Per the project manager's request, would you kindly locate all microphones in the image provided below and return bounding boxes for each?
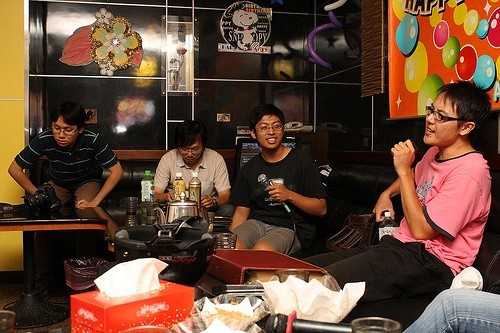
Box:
[264,311,353,333]
[258,174,291,212]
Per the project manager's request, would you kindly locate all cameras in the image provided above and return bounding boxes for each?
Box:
[20,183,62,214]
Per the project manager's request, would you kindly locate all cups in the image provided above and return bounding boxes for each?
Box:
[216,233,237,249]
[351,317,401,333]
[125,214,139,228]
[124,196,138,214]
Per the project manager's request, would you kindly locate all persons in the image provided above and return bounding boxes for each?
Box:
[301,80,492,305]
[154,120,236,217]
[228,104,328,256]
[402,288,500,333]
[8,105,123,295]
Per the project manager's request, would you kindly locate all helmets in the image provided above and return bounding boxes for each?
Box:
[114,216,215,287]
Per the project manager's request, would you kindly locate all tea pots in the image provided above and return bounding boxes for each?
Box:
[153,193,210,226]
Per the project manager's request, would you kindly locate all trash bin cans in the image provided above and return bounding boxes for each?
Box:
[65,258,108,299]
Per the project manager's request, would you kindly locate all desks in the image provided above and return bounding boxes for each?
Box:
[0,202,108,328]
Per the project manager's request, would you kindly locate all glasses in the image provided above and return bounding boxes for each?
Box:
[51,121,78,135]
[257,122,287,132]
[425,106,471,123]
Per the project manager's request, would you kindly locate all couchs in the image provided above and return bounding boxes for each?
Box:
[27,154,499,295]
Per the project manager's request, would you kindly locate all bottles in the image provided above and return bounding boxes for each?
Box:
[379,212,398,241]
[141,171,154,202]
[188,171,201,210]
[173,173,185,201]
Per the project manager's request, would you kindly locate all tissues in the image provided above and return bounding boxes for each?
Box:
[69,258,196,333]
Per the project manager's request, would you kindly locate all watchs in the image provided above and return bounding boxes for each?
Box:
[212,198,217,208]
